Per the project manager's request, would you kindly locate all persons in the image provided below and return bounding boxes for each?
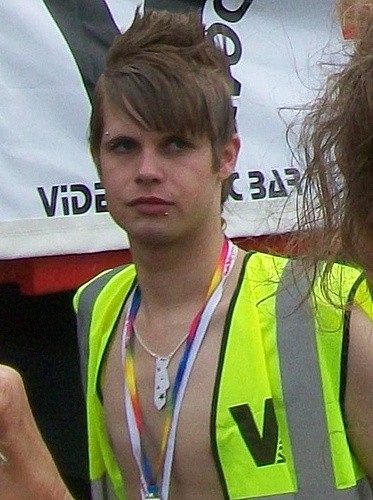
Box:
[0,0,373,500]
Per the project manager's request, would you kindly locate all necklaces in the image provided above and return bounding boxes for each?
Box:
[131,322,190,411]
